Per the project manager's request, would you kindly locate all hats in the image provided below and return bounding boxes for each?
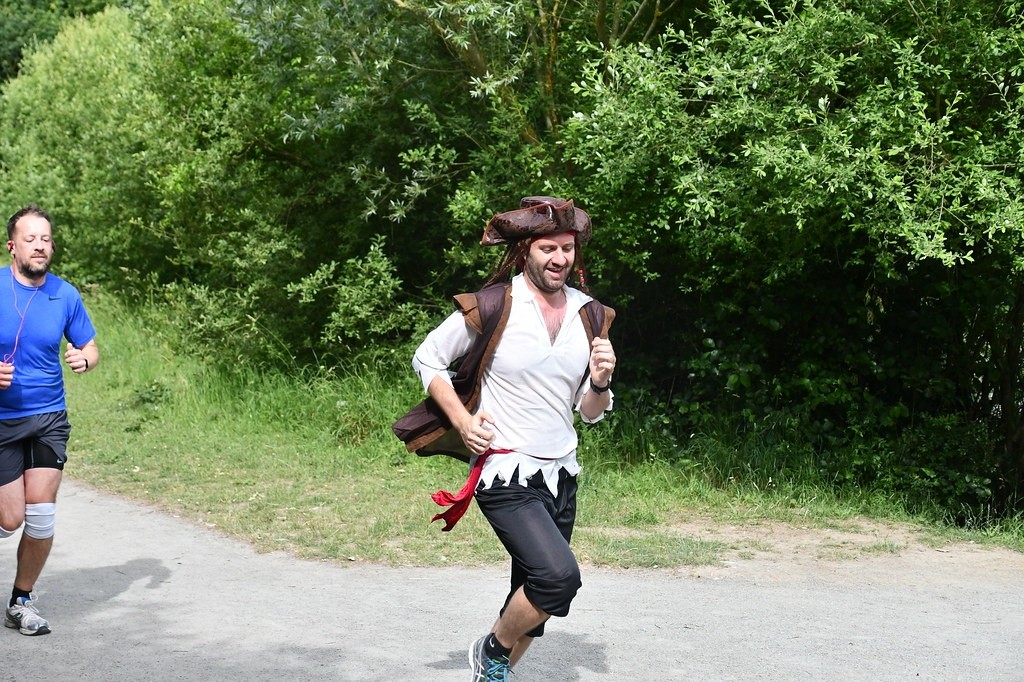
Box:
[479,196,592,247]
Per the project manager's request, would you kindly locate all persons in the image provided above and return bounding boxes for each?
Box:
[389,196,617,682]
[0,206,100,636]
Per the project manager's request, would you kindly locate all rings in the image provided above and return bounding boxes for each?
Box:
[477,438,481,446]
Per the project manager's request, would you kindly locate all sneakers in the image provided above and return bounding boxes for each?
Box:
[3,593,52,635]
[469,635,515,682]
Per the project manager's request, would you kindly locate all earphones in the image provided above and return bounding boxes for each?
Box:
[52,243,55,252]
[8,244,13,253]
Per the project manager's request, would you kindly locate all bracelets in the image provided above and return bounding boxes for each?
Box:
[589,376,611,394]
[83,358,88,372]
